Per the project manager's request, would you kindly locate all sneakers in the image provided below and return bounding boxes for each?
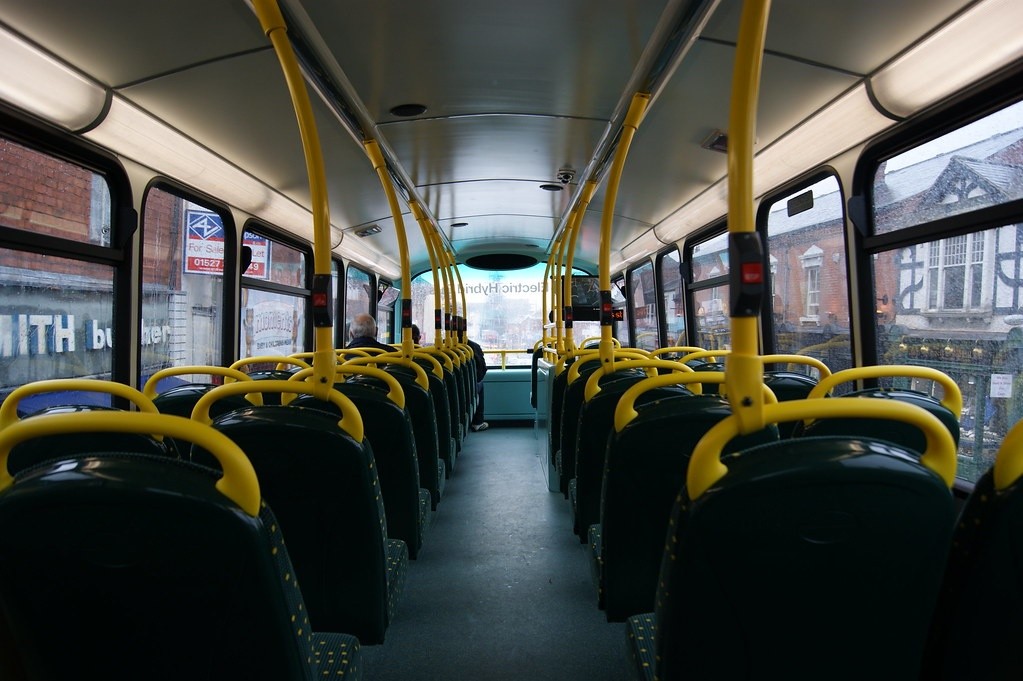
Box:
[471,422,488,433]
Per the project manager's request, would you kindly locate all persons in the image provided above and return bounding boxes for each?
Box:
[772,312,795,333]
[441,317,488,431]
[822,314,838,336]
[411,324,422,349]
[344,313,399,368]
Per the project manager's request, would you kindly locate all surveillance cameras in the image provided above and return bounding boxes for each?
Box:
[557,169,575,185]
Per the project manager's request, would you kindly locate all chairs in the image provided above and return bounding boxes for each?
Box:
[1,343,479,681]
[533,337,1023,680]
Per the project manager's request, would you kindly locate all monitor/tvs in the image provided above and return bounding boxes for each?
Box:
[562,275,600,308]
